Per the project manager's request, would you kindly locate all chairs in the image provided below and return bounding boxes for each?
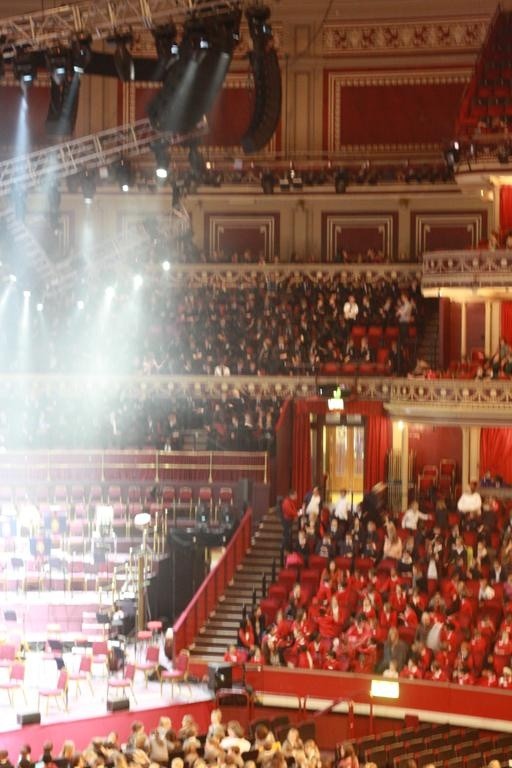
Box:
[170,283,421,375]
[228,554,511,691]
[283,500,509,558]
[0,481,232,592]
[417,461,456,499]
[0,611,193,717]
[427,347,512,382]
[338,723,512,767]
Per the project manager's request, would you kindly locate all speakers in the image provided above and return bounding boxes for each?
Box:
[147,45,232,132]
[208,663,231,689]
[241,48,281,154]
[107,698,129,710]
[46,79,80,135]
[17,712,40,724]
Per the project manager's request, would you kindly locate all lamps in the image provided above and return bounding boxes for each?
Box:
[445,138,510,171]
[1,245,176,318]
[165,161,452,194]
[10,133,208,221]
[1,2,274,93]
